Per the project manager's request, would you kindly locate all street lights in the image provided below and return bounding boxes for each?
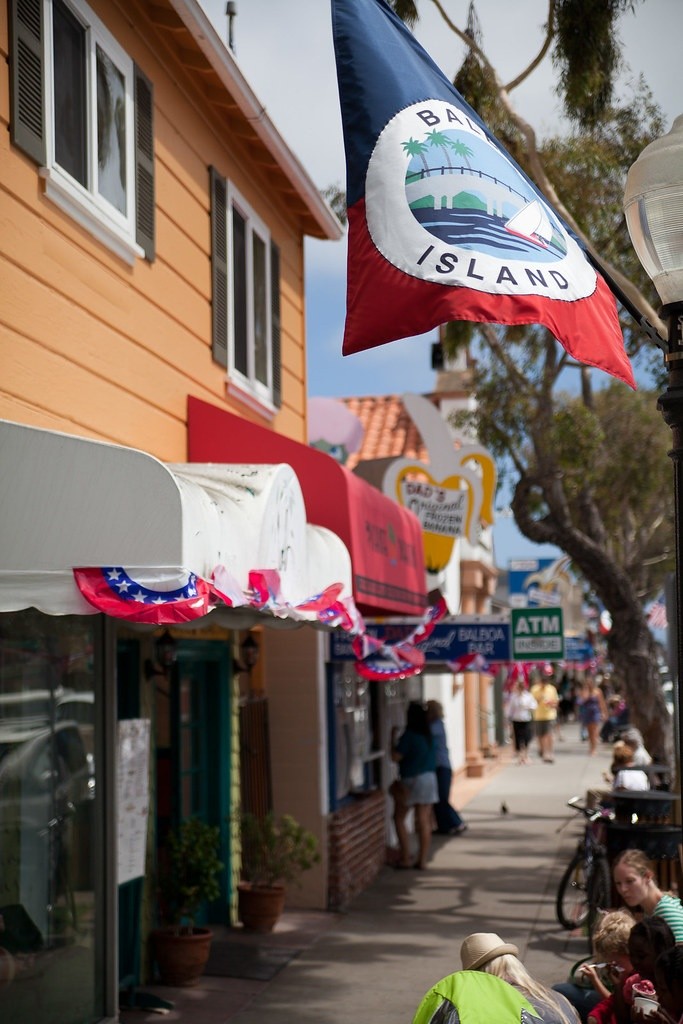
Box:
[624,108,683,900]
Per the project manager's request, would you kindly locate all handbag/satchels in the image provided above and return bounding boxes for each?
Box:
[389,780,412,801]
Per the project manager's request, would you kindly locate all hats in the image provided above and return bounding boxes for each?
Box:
[460,931,520,971]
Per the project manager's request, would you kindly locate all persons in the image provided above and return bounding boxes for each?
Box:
[588,908,636,1024]
[425,700,468,835]
[416,933,584,1024]
[616,916,675,1023]
[582,963,612,1002]
[587,728,659,825]
[507,666,629,763]
[631,945,683,1024]
[553,851,683,1022]
[391,700,439,871]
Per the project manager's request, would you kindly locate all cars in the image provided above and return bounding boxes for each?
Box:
[0,685,98,786]
[2,716,98,956]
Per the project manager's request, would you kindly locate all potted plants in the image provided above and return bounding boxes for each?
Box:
[236,810,324,937]
[149,817,229,989]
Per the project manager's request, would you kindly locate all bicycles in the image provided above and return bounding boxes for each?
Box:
[555,796,612,957]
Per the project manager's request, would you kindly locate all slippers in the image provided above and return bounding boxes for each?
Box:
[432,829,449,836]
[452,826,467,836]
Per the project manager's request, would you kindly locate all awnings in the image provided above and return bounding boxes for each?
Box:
[188,396,428,619]
[2,418,365,634]
[326,616,600,671]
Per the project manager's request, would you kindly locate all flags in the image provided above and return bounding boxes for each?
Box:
[332,1,640,390]
